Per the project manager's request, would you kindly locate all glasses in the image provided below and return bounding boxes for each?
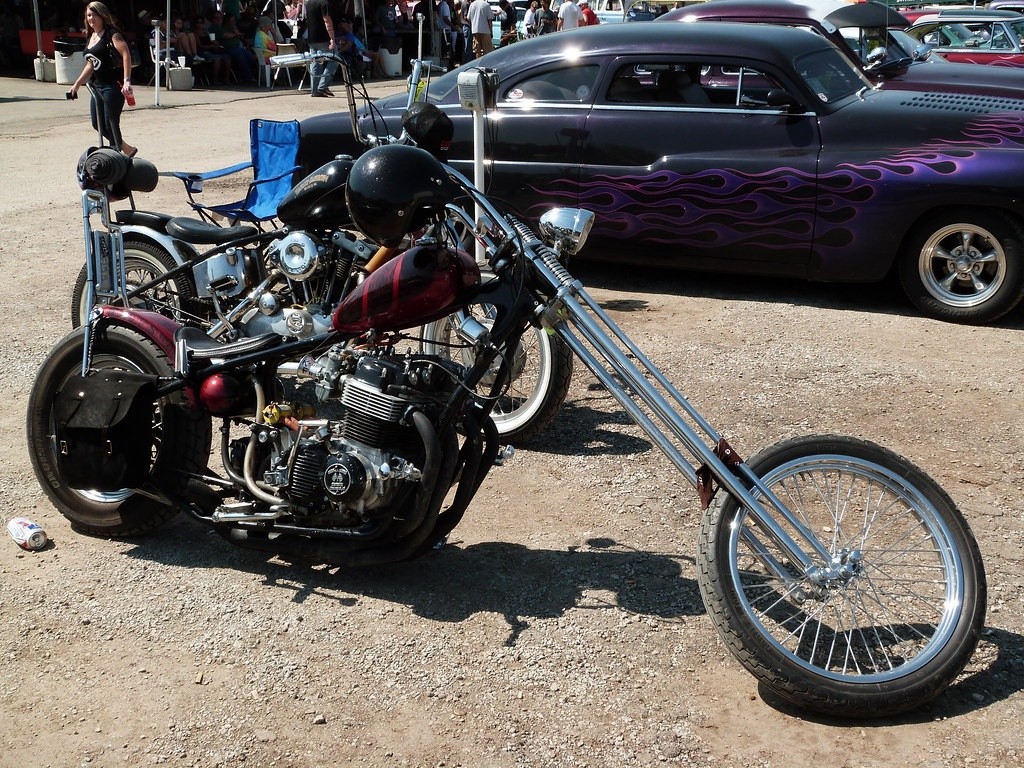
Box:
[195,21,203,24]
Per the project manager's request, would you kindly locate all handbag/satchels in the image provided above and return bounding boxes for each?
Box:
[52,369,159,492]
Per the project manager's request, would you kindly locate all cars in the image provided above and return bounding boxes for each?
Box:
[286,0,1024,326]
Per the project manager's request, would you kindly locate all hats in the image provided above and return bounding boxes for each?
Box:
[258,16,273,27]
[138,10,150,24]
[576,0,588,6]
[403,102,454,158]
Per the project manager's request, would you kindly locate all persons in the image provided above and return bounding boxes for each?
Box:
[492,0,519,48]
[306,0,405,97]
[69,1,138,158]
[138,0,293,86]
[522,0,600,39]
[412,0,496,72]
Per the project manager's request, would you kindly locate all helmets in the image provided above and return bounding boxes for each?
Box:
[345,144,452,248]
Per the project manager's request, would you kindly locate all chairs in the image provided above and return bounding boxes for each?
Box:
[252,47,292,87]
[658,71,710,104]
[609,78,642,99]
[173,117,305,235]
[147,46,179,86]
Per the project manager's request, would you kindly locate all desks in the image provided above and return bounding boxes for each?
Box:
[270,60,311,91]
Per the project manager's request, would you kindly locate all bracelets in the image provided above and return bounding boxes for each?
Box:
[123,78,131,80]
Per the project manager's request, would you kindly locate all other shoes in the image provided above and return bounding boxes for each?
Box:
[312,93,328,97]
[193,55,204,61]
[320,88,334,96]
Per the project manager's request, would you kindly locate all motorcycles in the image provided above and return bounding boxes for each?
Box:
[25,50,987,720]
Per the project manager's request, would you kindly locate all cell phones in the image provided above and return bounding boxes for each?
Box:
[66,92,78,99]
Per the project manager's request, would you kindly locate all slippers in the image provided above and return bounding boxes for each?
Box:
[129,147,138,158]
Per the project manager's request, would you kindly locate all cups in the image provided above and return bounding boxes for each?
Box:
[209,34,215,41]
[121,91,136,106]
[178,57,185,68]
[286,38,290,44]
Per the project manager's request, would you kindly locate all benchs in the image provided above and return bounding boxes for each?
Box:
[17,26,137,68]
